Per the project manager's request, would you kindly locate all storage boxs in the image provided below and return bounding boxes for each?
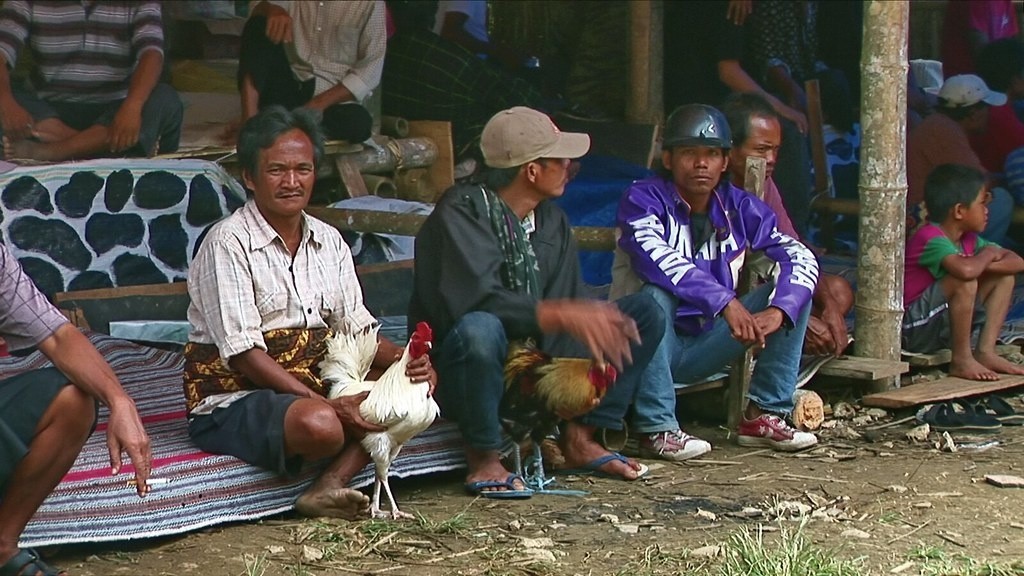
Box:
[172,15,246,58]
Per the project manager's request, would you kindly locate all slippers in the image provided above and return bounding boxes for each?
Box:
[465,473,533,498]
[970,394,1024,426]
[914,398,1004,434]
[560,452,650,482]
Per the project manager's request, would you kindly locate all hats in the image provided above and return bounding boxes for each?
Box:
[936,74,1009,108]
[479,105,592,170]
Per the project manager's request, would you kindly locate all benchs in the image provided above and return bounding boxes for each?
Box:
[0,58,750,547]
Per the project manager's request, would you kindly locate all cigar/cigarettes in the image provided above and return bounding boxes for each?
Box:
[127,478,174,485]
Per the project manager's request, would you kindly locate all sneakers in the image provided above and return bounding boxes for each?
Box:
[736,411,818,451]
[638,429,712,462]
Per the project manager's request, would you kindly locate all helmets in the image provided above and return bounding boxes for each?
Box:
[662,103,734,150]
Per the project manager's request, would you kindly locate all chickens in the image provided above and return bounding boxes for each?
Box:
[498,334,617,491]
[317,318,440,522]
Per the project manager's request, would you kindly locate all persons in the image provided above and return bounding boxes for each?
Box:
[0,0,611,164]
[609,107,854,461]
[0,240,151,576]
[183,104,437,519]
[408,107,642,497]
[701,0,1024,381]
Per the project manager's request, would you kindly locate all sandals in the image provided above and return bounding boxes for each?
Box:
[1,547,68,576]
